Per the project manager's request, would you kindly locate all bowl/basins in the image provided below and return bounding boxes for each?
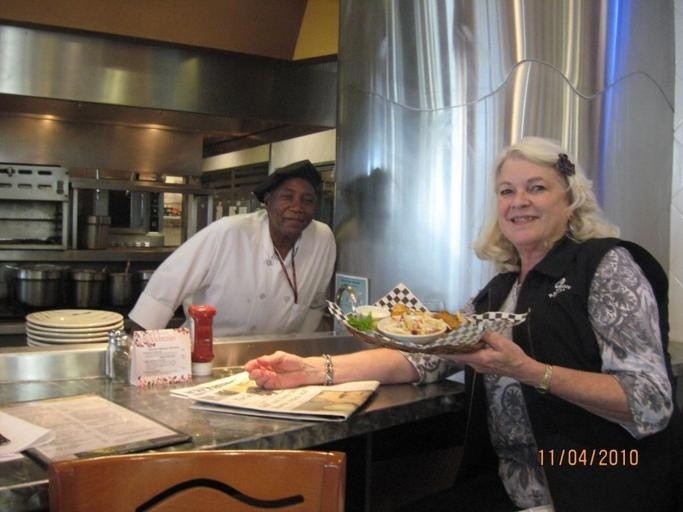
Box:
[375,315,448,344]
[354,305,390,322]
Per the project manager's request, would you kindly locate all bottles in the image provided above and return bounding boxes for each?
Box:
[187,303,216,378]
[104,328,130,391]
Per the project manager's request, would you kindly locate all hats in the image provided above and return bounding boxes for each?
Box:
[253,160,322,204]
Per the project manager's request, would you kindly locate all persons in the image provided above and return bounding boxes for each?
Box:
[128,158,338,338]
[245,136,683,511]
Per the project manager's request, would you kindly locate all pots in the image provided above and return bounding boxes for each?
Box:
[0,255,155,309]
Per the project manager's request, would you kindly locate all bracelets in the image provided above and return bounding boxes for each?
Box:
[323,354,333,385]
[535,364,553,394]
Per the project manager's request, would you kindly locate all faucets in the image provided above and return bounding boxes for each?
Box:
[334,283,358,314]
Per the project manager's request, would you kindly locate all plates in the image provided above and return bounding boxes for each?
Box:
[342,323,489,354]
[23,308,124,347]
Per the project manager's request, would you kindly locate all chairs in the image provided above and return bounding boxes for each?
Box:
[48,449,347,512]
